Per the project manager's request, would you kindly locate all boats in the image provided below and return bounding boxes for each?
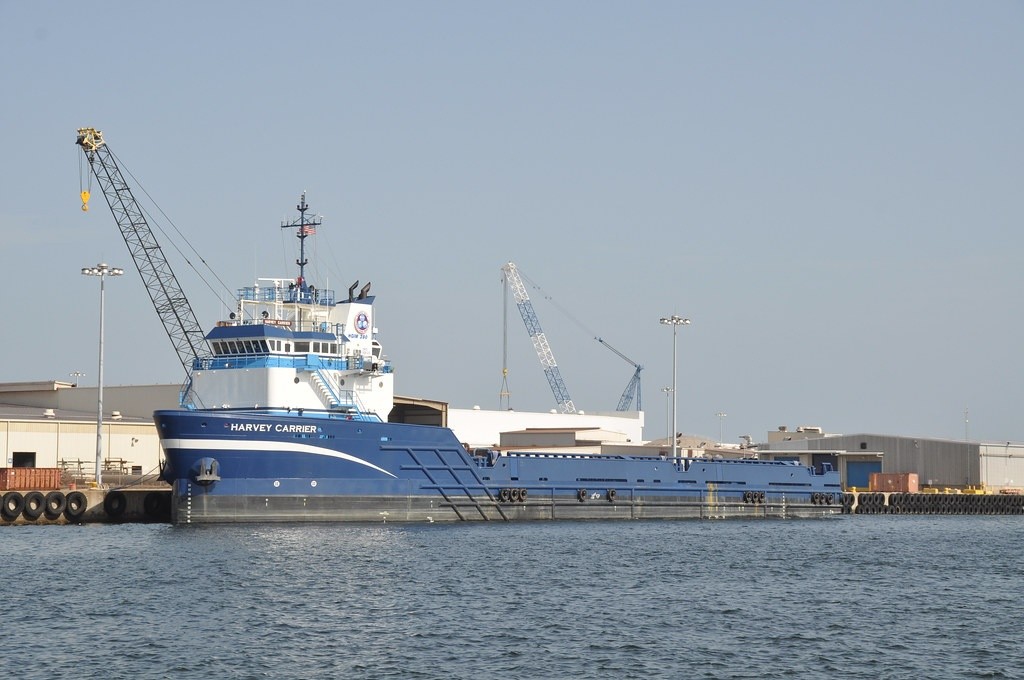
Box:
[153,190,854,527]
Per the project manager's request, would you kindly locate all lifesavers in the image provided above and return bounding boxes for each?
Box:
[742,490,766,503]
[499,488,528,502]
[103,490,128,518]
[840,492,1024,516]
[0,491,89,521]
[577,488,588,502]
[607,488,618,502]
[143,491,167,516]
[811,492,834,505]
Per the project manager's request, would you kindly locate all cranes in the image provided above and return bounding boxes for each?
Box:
[72,124,223,369]
[495,260,645,417]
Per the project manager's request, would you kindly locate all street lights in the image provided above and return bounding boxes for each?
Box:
[80,263,124,487]
[661,384,674,443]
[656,313,691,457]
[715,411,727,446]
